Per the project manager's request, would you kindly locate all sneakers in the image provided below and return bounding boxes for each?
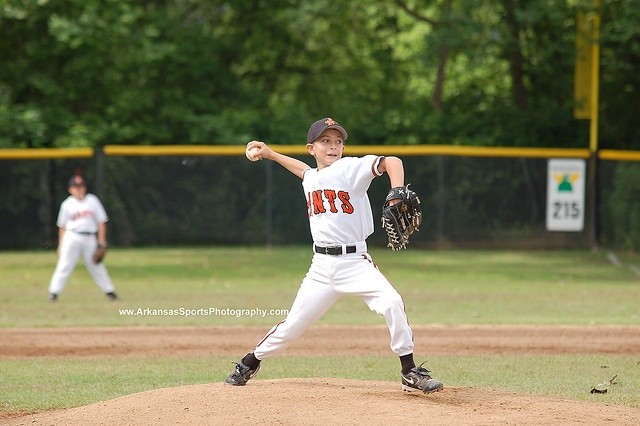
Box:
[47,294,58,304]
[402,360,444,394]
[224,353,260,387]
[107,292,119,302]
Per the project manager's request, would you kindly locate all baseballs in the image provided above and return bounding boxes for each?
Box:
[245,147,261,162]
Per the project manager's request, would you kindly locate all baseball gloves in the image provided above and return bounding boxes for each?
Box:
[94,244,106,263]
[382,183,422,251]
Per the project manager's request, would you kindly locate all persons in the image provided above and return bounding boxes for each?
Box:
[47,174,123,301]
[225,119,444,395]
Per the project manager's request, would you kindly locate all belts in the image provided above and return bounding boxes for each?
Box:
[72,232,98,235]
[312,245,358,256]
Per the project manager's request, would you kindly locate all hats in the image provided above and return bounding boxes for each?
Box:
[68,174,86,188]
[307,118,347,145]
